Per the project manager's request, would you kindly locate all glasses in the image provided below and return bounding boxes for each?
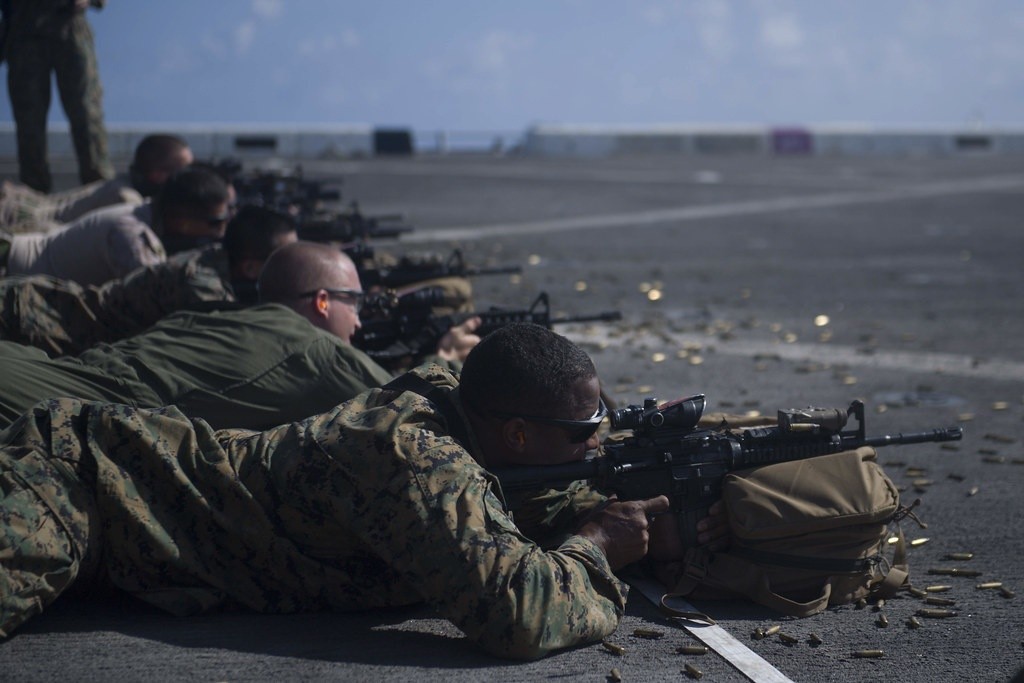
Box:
[299,289,365,313]
[180,211,230,231]
[489,395,606,444]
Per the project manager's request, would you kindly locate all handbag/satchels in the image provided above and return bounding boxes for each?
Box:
[599,404,916,619]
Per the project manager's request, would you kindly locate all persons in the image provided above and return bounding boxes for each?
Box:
[0,0,671,664]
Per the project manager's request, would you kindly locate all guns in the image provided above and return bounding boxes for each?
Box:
[350,286,621,373]
[293,207,415,245]
[342,243,524,291]
[486,391,965,558]
